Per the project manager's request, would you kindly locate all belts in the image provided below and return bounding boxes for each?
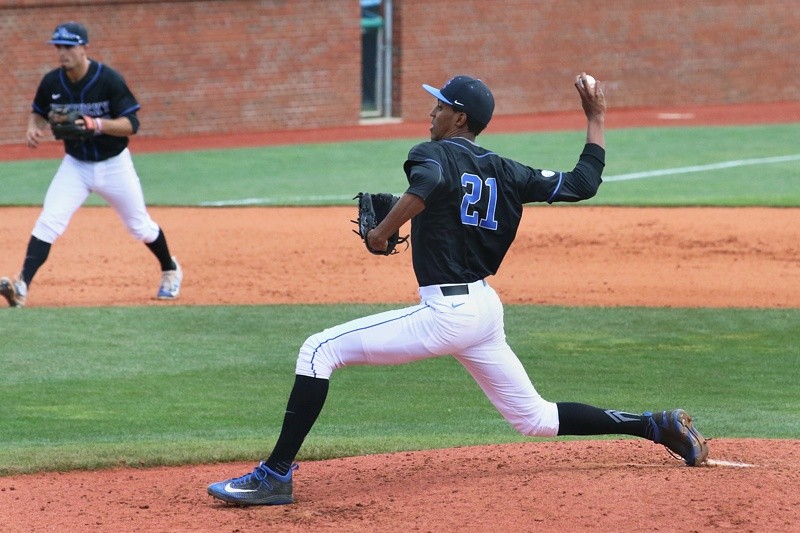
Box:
[441,280,488,295]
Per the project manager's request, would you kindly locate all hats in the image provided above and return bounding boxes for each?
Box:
[47,22,88,45]
[422,75,495,124]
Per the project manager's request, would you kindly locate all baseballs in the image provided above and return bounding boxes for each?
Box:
[579,75,596,89]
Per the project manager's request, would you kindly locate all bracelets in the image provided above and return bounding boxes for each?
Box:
[95,118,102,136]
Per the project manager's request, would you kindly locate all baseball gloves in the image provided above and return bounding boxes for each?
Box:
[48,111,97,146]
[349,192,410,257]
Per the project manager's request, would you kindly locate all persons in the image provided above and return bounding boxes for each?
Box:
[207,71,710,508]
[1,21,183,308]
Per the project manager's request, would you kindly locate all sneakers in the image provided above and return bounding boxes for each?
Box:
[651,409,709,467]
[0,277,27,308]
[157,256,182,298]
[207,462,299,504]
